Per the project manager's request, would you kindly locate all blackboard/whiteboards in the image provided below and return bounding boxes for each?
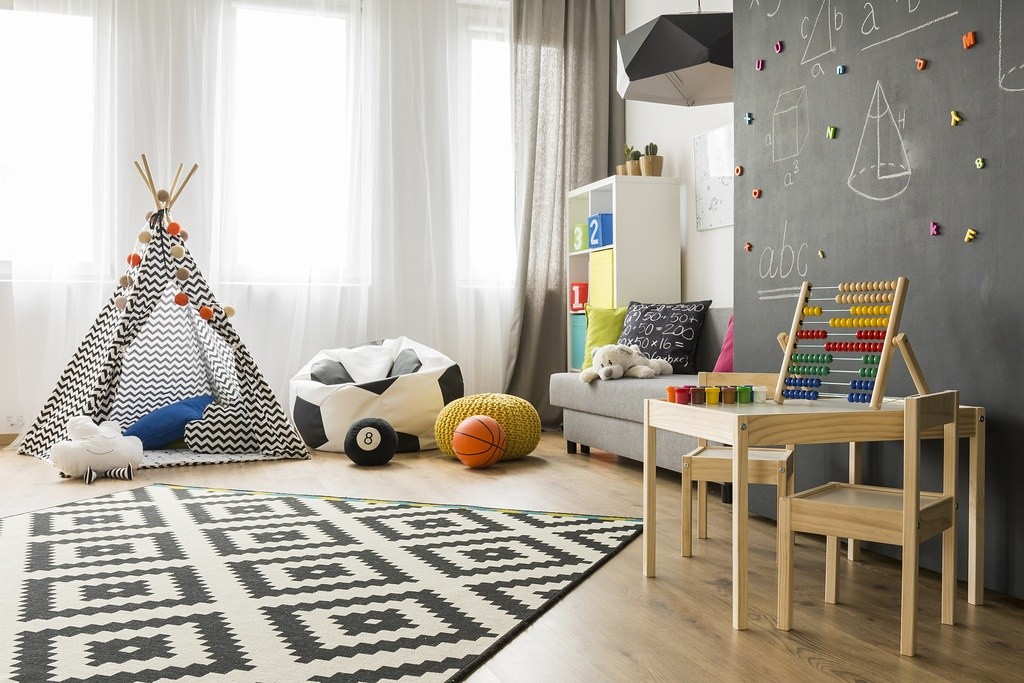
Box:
[692,122,734,231]
[733,0,1024,602]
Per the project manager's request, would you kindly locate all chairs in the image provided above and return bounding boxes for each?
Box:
[776,388,960,658]
[682,371,794,559]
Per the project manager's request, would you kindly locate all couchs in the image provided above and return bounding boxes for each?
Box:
[549,299,733,503]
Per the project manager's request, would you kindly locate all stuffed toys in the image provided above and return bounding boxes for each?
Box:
[49,416,144,484]
[579,344,672,385]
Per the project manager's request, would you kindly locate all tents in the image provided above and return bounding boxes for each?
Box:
[19,154,311,484]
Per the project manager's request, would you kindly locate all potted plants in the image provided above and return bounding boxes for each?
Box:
[615,145,635,175]
[626,151,641,175]
[640,143,664,176]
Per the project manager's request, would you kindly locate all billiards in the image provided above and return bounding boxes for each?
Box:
[343,419,397,467]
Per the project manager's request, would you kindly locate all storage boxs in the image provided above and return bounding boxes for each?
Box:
[573,224,588,252]
[570,282,588,311]
[587,212,611,248]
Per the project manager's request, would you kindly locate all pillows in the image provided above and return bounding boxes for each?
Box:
[581,302,627,371]
[617,299,712,374]
[123,394,216,449]
[712,310,733,372]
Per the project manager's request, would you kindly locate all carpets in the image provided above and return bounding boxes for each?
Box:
[0,482,658,683]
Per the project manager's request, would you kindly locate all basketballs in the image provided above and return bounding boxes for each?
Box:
[452,415,507,467]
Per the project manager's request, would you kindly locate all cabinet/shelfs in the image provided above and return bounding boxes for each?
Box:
[565,175,681,372]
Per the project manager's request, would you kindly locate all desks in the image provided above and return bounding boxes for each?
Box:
[642,394,985,632]
